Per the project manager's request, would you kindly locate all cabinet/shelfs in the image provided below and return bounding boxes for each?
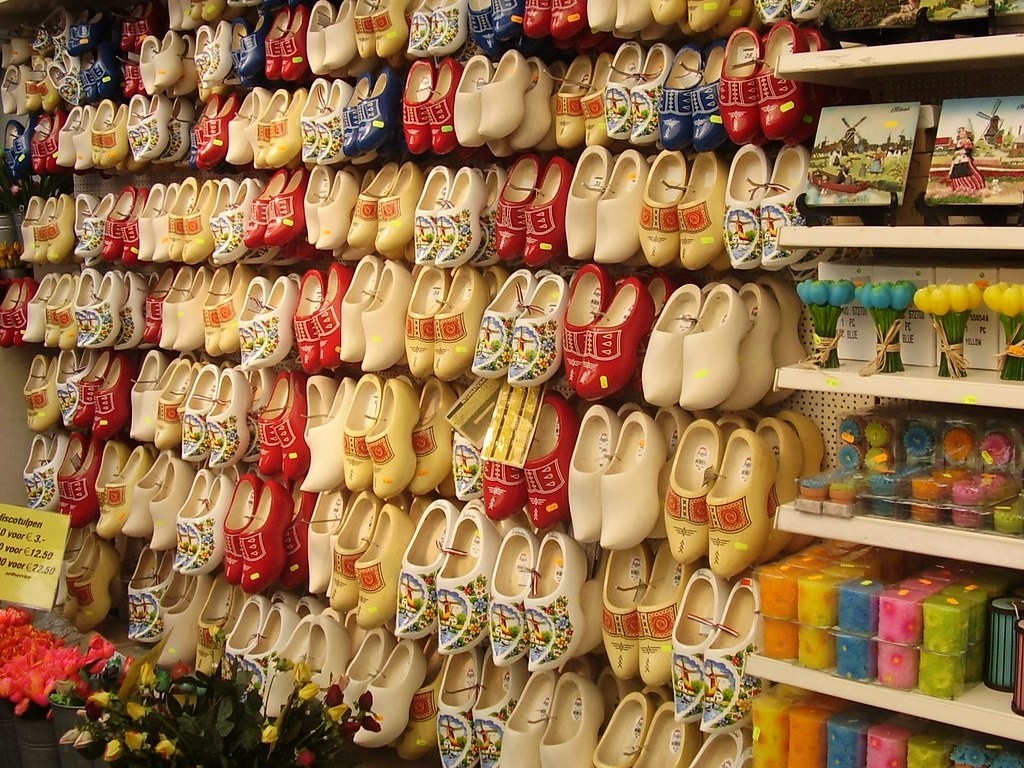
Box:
[736,29,1024,753]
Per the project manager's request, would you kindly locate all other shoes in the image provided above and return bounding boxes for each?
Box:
[0,0,826,768]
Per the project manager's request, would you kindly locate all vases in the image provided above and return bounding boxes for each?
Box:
[12,699,58,768]
[0,696,22,768]
[49,697,105,768]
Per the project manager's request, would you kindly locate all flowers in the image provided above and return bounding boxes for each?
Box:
[0,603,384,768]
